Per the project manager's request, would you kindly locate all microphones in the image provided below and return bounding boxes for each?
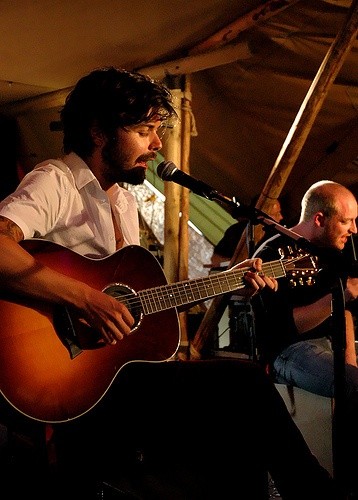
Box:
[157,160,218,201]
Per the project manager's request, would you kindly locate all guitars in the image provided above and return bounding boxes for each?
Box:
[0,239,322,424]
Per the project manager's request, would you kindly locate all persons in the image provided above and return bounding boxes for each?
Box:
[0,65,334,500]
[249,180,358,398]
[211,194,258,265]
